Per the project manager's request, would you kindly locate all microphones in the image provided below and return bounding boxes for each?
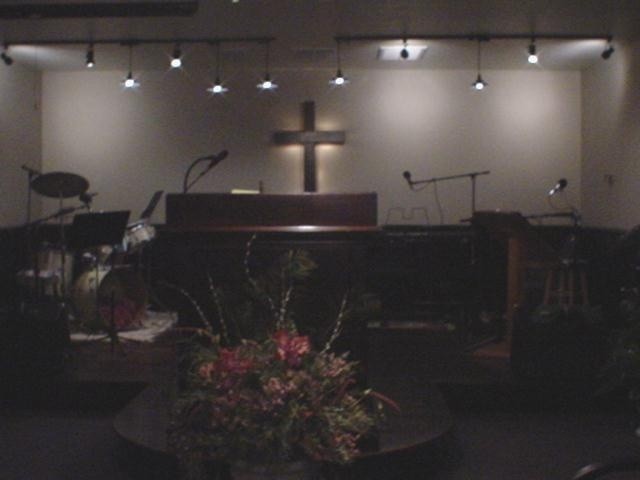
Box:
[403,171,414,189]
[259,180,264,194]
[21,164,41,176]
[79,191,93,209]
[548,178,568,196]
[208,150,229,168]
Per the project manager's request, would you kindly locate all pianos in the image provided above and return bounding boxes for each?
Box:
[155,193,383,353]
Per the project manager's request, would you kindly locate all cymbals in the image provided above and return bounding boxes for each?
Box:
[30,172,88,198]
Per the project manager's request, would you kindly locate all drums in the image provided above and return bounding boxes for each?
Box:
[125,219,155,250]
[70,267,136,331]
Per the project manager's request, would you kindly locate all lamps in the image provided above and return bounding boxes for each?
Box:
[0,35,282,93]
[324,30,617,91]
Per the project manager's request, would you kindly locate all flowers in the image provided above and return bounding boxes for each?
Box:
[171,327,379,467]
